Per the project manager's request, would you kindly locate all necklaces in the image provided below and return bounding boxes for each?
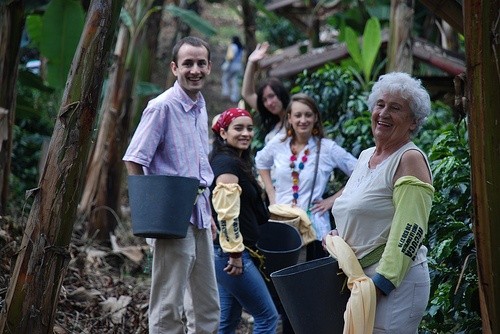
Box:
[290,146,310,205]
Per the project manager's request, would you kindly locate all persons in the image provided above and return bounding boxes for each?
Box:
[208,108,279,334]
[254,92,358,259]
[322,72,435,334]
[242,41,292,145]
[122,36,222,334]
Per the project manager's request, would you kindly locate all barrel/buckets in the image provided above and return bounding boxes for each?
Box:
[253,221,303,284]
[270,256,351,334]
[128,174,200,238]
